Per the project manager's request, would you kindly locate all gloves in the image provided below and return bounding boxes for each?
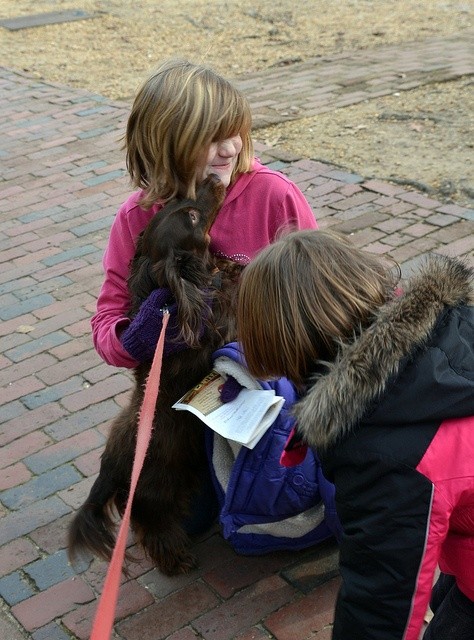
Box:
[219,375,244,403]
[120,286,216,363]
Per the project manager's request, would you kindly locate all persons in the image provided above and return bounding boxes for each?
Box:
[91,62,318,370]
[235,218,473,640]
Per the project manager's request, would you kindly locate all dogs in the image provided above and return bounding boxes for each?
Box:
[66,173,237,583]
